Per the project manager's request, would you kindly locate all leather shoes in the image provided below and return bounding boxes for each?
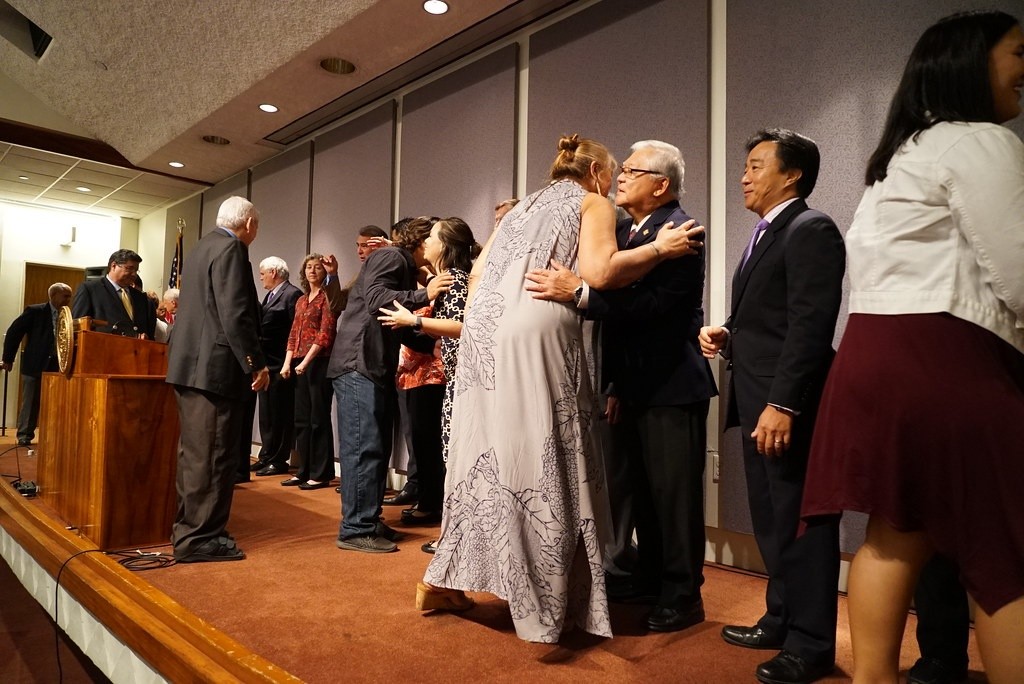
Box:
[754,650,834,683]
[401,508,417,517]
[256,462,289,475]
[299,480,329,490]
[401,511,441,525]
[422,540,437,553]
[281,479,306,486]
[382,490,415,505]
[642,595,706,633]
[721,625,782,649]
[605,580,660,605]
[250,460,268,470]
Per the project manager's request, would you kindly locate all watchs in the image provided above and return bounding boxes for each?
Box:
[573,287,584,303]
[412,317,423,335]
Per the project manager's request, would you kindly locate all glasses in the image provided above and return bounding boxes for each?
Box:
[620,165,661,176]
[115,261,141,275]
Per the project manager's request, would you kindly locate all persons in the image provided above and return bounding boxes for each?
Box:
[0,282,71,446]
[166,196,271,563]
[525,139,720,632]
[698,130,847,684]
[319,225,388,493]
[72,249,156,341]
[131,273,180,344]
[250,255,304,476]
[326,221,455,553]
[796,12,1024,684]
[280,253,336,490]
[493,198,520,229]
[366,217,483,554]
[416,134,705,644]
[599,192,638,578]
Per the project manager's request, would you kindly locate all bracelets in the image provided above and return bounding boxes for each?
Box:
[647,241,662,262]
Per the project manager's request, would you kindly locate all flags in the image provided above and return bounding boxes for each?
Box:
[168,232,185,289]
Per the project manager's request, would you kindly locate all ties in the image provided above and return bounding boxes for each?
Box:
[268,292,274,302]
[625,229,637,249]
[120,288,133,320]
[738,218,770,277]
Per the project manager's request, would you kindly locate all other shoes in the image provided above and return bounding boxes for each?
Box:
[179,537,245,562]
[336,533,398,553]
[904,662,968,683]
[375,521,404,541]
[18,439,32,446]
[234,473,250,484]
[335,486,341,493]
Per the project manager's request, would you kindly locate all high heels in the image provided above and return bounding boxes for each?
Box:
[415,583,474,611]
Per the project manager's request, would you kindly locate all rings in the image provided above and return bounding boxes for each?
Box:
[774,440,782,443]
